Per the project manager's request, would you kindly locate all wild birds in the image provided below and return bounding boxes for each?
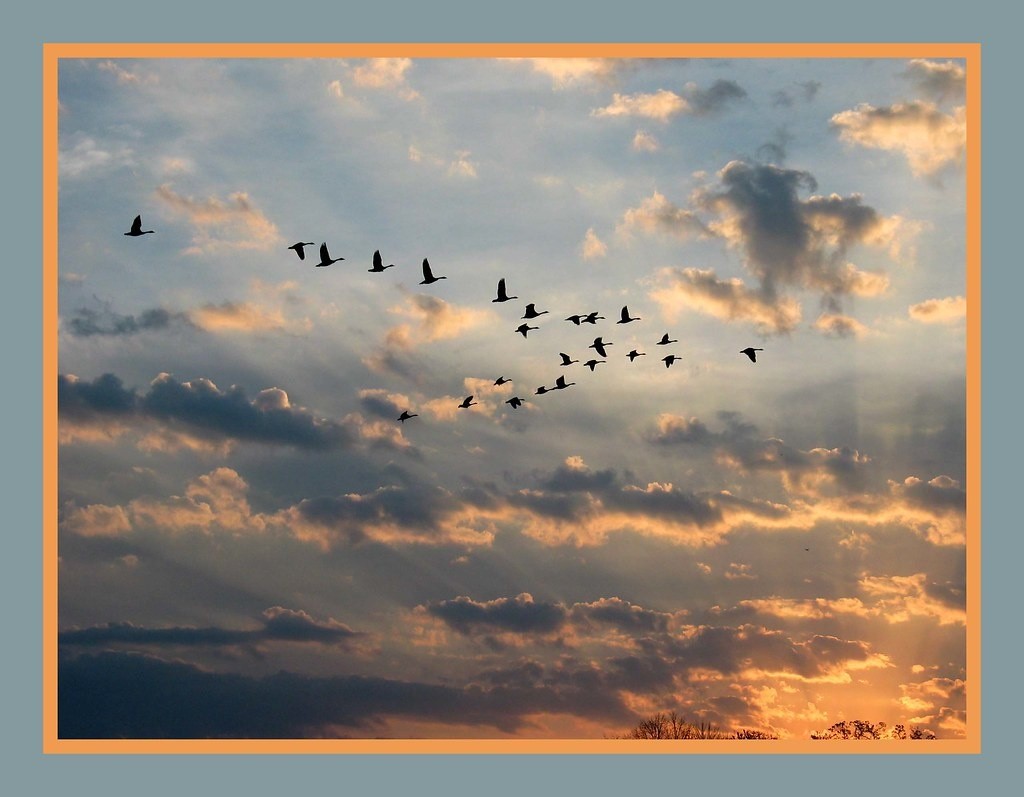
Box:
[492,277,764,371]
[124,215,155,237]
[288,242,447,285]
[397,375,576,425]
